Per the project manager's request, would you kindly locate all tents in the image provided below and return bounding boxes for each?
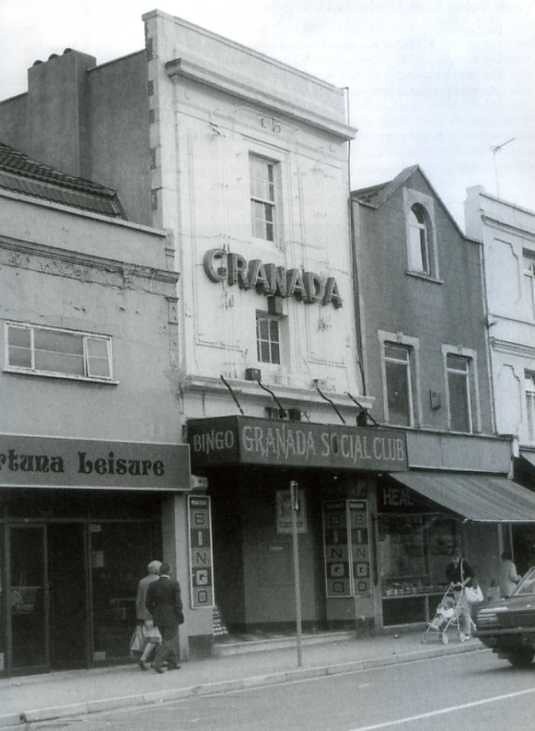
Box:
[388,470,534,526]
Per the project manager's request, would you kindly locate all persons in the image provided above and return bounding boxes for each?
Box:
[426,592,457,632]
[497,551,521,600]
[134,560,184,674]
[444,547,476,640]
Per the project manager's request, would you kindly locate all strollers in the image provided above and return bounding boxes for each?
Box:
[421,581,468,645]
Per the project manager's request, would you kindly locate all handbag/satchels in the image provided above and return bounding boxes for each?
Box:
[465,584,484,604]
[130,624,145,652]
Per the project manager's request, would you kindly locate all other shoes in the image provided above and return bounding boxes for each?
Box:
[168,664,180,670]
[151,663,164,673]
[464,635,470,640]
[139,659,148,670]
[160,663,166,667]
[472,624,477,631]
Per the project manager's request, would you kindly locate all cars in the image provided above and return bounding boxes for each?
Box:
[470,564,535,670]
